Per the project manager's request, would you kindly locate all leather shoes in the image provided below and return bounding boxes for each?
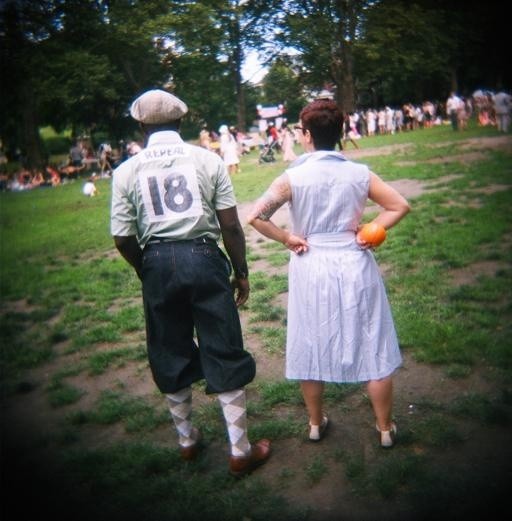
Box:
[177,428,204,462]
[228,438,272,478]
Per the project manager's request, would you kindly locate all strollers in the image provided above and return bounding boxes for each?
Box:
[256,139,279,163]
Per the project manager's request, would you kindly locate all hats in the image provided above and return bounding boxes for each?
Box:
[130,88,189,125]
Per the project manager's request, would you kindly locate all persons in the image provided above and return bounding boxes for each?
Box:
[246,99,413,449]
[107,90,271,473]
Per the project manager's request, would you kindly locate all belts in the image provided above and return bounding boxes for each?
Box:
[145,233,217,247]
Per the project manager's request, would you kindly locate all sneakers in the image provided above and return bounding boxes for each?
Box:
[308,416,328,443]
[375,418,399,449]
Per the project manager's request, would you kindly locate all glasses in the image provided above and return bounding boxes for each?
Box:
[292,124,305,131]
